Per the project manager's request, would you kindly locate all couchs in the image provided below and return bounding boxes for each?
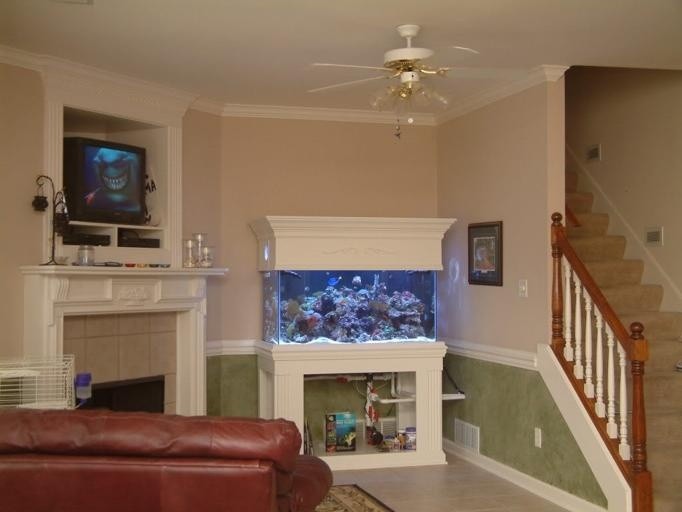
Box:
[1,407,333,512]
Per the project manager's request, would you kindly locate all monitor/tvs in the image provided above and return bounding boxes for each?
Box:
[63,137,145,225]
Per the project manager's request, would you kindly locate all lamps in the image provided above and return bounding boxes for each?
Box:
[369,69,450,139]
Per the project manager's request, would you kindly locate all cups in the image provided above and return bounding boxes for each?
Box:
[183,232,216,268]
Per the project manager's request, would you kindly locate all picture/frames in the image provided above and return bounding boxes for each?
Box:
[468,221,502,286]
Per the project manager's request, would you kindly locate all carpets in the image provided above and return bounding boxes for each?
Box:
[315,483,395,512]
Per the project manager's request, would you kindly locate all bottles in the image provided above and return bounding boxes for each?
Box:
[390,437,401,454]
[77,243,95,266]
[406,427,416,451]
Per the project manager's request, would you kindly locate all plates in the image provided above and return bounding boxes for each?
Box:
[125,263,171,268]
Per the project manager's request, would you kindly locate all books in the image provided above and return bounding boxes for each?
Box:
[326,412,357,452]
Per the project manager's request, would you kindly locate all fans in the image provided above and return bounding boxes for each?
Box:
[307,24,515,99]
